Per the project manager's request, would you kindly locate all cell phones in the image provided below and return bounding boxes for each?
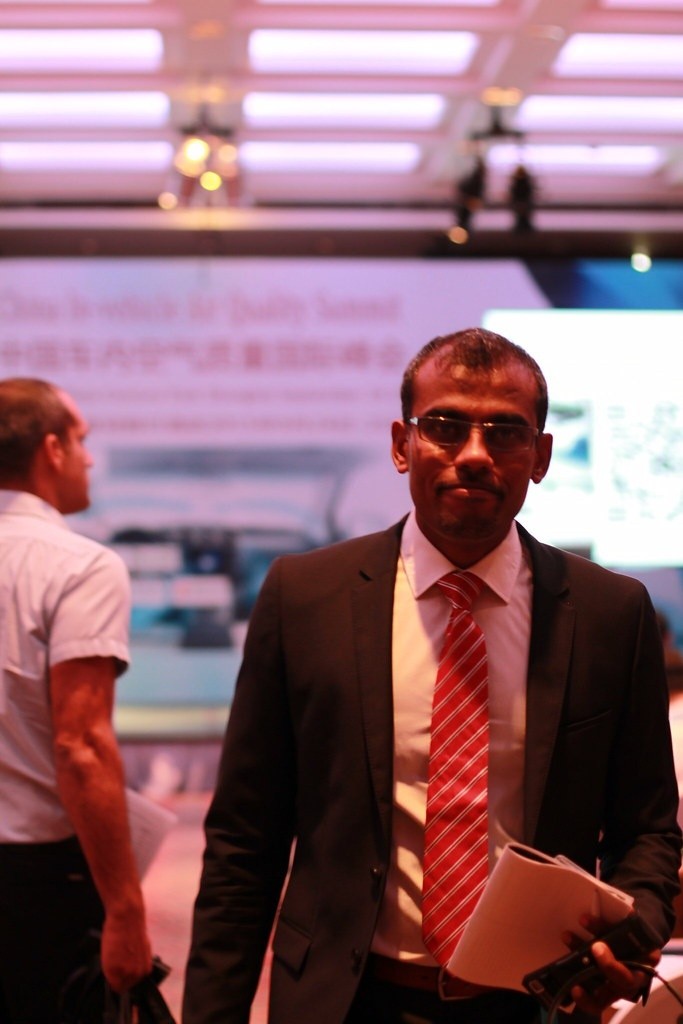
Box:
[523,914,664,1012]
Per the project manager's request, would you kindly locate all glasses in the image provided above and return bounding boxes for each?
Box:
[403,415,541,454]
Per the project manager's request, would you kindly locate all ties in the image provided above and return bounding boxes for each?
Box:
[420,572,492,977]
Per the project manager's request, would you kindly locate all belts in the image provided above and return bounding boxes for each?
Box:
[363,951,497,1001]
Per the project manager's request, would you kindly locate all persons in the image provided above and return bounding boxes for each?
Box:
[184,328,683,1024]
[0,376,179,1024]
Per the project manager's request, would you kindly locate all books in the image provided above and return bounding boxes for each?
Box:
[443,840,633,994]
